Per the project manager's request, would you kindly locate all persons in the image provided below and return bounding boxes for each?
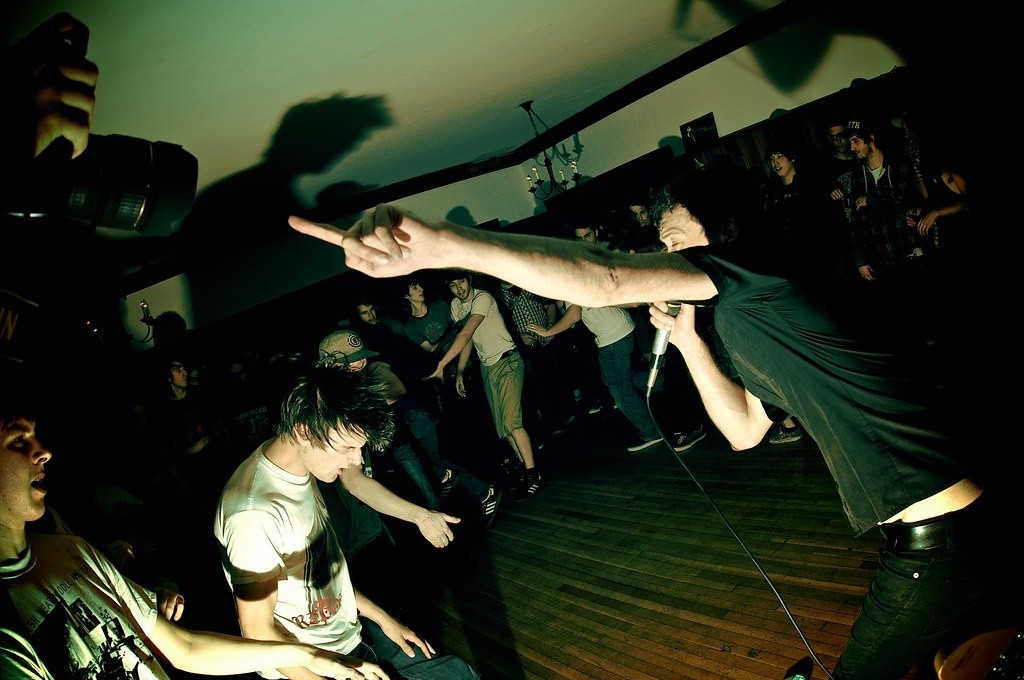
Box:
[0,329,392,680]
[96,101,992,621]
[213,329,482,680]
[288,182,1024,680]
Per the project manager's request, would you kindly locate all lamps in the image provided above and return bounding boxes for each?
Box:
[517,100,583,200]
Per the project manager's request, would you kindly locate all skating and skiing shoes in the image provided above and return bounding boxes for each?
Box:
[623,433,663,451]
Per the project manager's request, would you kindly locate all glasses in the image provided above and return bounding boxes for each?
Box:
[900,210,922,228]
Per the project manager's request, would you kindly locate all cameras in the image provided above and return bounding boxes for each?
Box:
[0,117,199,237]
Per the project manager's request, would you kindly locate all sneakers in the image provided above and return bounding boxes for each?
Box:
[511,466,543,502]
[481,485,502,530]
[674,422,706,452]
[766,425,801,445]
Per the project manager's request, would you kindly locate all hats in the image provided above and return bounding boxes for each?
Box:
[313,329,382,370]
[831,115,879,142]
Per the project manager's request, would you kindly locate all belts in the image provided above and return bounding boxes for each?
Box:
[499,349,514,360]
[867,498,999,549]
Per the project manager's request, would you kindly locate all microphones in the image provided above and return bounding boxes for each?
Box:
[645,301,683,398]
